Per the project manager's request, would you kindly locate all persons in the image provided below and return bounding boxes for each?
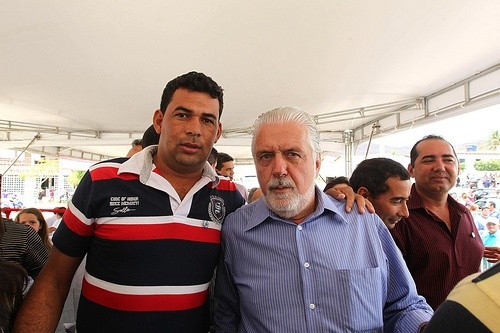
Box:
[349,158,412,229]
[390,135,486,311]
[215,106,435,333]
[0,124,264,333]
[423,261,500,333]
[446,171,500,263]
[10,72,374,333]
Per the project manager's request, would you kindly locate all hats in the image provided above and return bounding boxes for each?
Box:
[485,217,499,225]
